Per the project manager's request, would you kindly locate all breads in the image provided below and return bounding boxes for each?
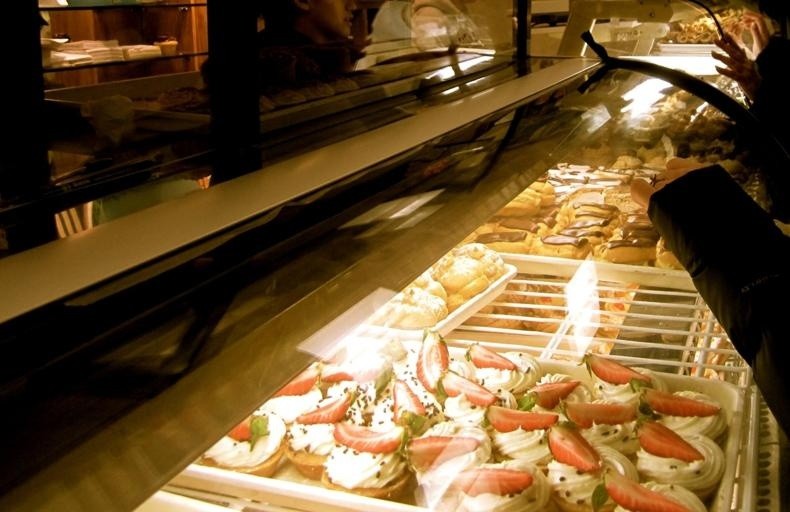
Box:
[158,65,401,112]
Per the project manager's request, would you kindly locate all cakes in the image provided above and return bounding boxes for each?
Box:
[583,92,744,181]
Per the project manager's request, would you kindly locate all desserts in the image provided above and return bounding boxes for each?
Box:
[372,161,749,329]
[203,333,727,512]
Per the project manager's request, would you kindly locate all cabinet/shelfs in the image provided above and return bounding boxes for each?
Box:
[0,0,790,512]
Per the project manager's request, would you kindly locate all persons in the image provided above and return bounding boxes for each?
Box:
[197,1,375,138]
[633,157,790,446]
[401,0,485,100]
[713,2,790,233]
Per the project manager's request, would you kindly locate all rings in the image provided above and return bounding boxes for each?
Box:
[649,174,664,188]
